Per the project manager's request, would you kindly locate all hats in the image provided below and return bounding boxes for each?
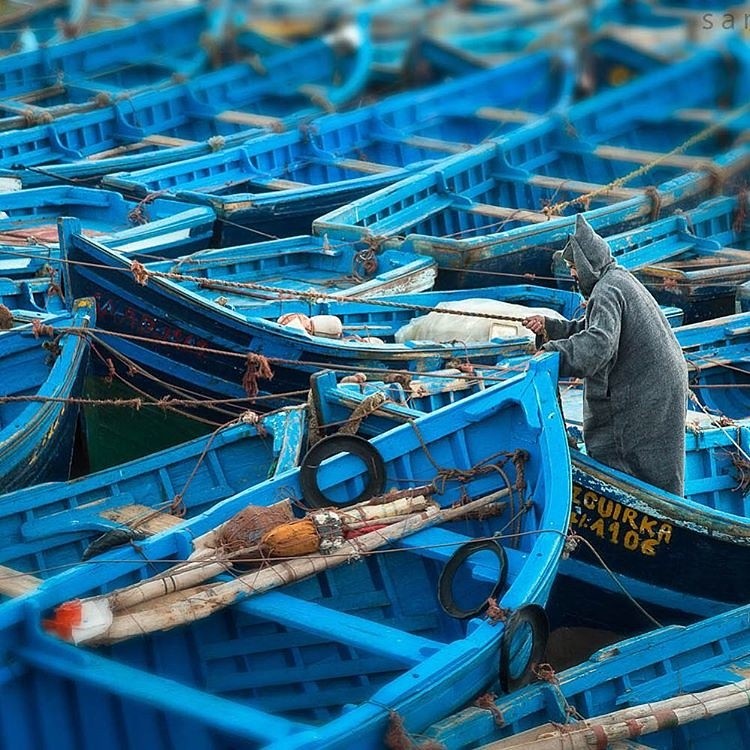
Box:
[561,241,574,262]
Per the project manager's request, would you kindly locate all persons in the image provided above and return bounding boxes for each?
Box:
[522,213,689,498]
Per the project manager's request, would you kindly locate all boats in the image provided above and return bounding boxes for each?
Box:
[0,2,750,750]
